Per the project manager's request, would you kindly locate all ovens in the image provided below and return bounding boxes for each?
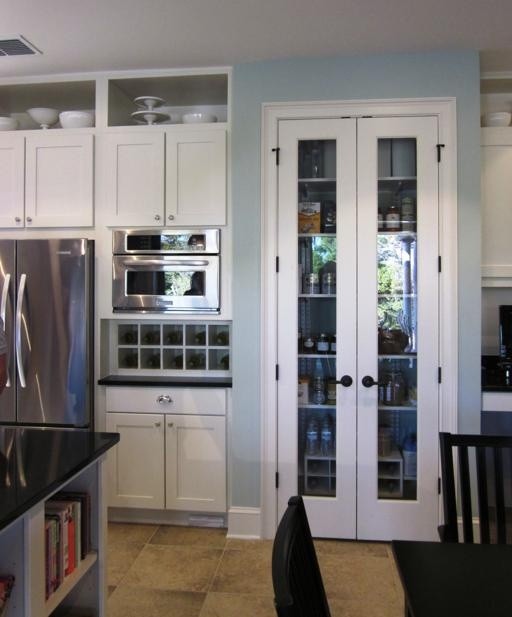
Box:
[111,226,221,316]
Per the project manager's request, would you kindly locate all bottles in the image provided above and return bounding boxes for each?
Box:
[165,331,183,346]
[321,271,336,294]
[122,353,138,368]
[220,355,229,370]
[307,415,336,457]
[172,354,183,368]
[216,330,229,345]
[302,273,320,293]
[194,331,205,345]
[188,353,205,369]
[120,330,138,344]
[142,331,160,345]
[147,354,160,368]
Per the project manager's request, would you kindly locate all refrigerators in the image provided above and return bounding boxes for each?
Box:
[0,239,96,428]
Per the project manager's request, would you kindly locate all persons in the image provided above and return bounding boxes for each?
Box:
[184,271,204,296]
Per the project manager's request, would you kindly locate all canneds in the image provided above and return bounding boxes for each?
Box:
[330,334,336,354]
[303,337,315,352]
[313,375,325,404]
[316,333,329,354]
[326,378,336,405]
[384,205,401,231]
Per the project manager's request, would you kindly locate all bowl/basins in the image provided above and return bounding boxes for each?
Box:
[0,116,18,130]
[58,109,95,128]
[182,113,218,123]
[10,112,34,129]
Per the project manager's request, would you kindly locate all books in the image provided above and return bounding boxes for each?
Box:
[46,491,93,601]
[0,575,16,617]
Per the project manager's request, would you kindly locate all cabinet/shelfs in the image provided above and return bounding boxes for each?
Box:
[0,452,109,617]
[102,386,230,529]
[260,97,459,545]
[0,72,100,239]
[107,320,232,377]
[102,65,233,230]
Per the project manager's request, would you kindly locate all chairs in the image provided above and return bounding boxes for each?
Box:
[272,496,332,617]
[438,432,512,543]
